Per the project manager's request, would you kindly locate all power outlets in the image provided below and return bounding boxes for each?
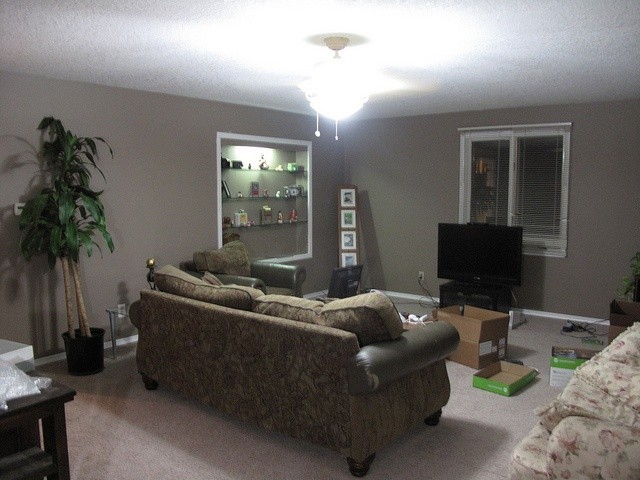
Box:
[419,272,424,281]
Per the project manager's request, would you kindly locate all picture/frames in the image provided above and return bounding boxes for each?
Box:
[338,183,360,272]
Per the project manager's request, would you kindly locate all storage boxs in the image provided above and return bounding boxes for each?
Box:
[432,305,510,368]
[550,346,599,388]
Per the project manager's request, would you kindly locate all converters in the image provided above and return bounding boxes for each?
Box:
[563,322,574,333]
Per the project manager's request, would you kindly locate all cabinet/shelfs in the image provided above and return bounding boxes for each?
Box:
[0,351,76,480]
[215,130,313,262]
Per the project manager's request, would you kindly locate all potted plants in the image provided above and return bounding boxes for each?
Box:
[6,121,111,371]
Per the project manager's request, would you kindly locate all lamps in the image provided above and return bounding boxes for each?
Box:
[293,34,370,140]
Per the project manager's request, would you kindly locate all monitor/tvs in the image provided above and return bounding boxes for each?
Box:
[327,264,364,298]
[436,222,523,288]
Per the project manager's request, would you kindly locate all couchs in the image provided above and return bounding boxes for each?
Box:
[129,263,459,477]
[509,323,640,479]
[180,241,306,298]
